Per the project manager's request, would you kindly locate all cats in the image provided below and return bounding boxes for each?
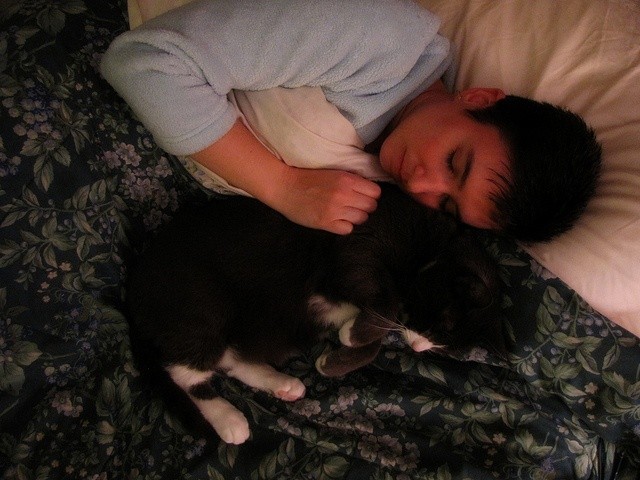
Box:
[122,183,501,445]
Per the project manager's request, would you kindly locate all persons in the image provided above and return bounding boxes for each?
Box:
[101,0,601,246]
